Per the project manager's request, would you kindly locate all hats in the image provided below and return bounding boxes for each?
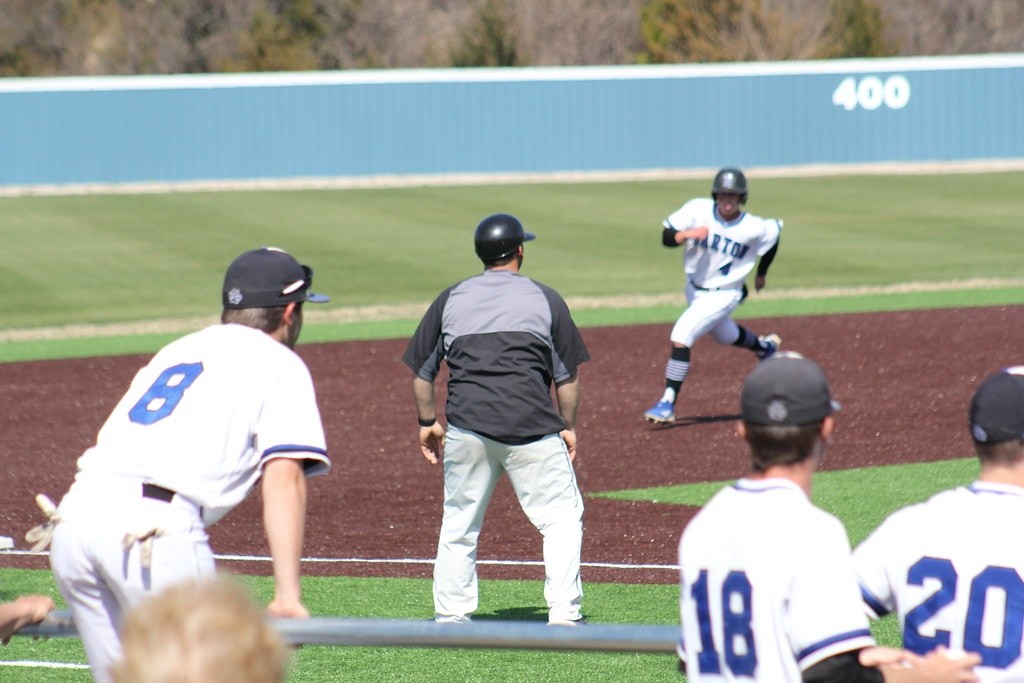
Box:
[970,364,1024,439]
[741,351,841,425]
[474,214,536,260]
[711,169,748,204]
[222,246,329,309]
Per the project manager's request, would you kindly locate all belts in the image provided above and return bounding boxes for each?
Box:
[690,280,743,292]
[69,476,203,521]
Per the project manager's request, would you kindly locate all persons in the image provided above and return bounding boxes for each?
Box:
[644,168,781,425]
[679,350,985,683]
[400,213,591,628]
[850,364,1024,683]
[33,244,331,683]
[0,595,57,645]
[107,575,292,683]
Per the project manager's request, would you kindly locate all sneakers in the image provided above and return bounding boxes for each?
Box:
[643,403,678,425]
[756,332,782,367]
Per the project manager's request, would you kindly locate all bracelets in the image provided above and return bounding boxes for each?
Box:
[418,415,436,427]
